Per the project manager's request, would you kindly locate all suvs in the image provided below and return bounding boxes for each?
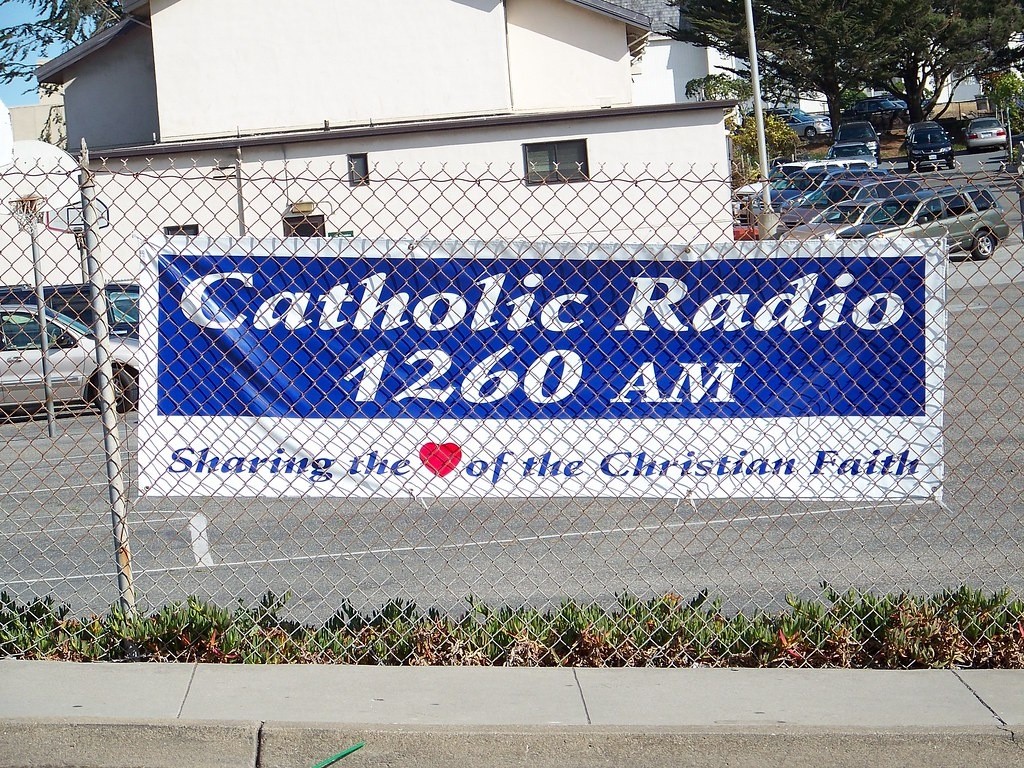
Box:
[775,180,925,236]
[731,157,873,214]
[740,167,887,224]
[836,182,1010,260]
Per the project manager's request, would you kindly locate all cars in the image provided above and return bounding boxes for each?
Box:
[1,284,141,338]
[779,197,888,240]
[836,95,936,125]
[905,121,945,140]
[0,305,139,413]
[904,128,957,171]
[835,121,884,165]
[825,142,879,173]
[743,106,832,137]
[960,117,1009,150]
